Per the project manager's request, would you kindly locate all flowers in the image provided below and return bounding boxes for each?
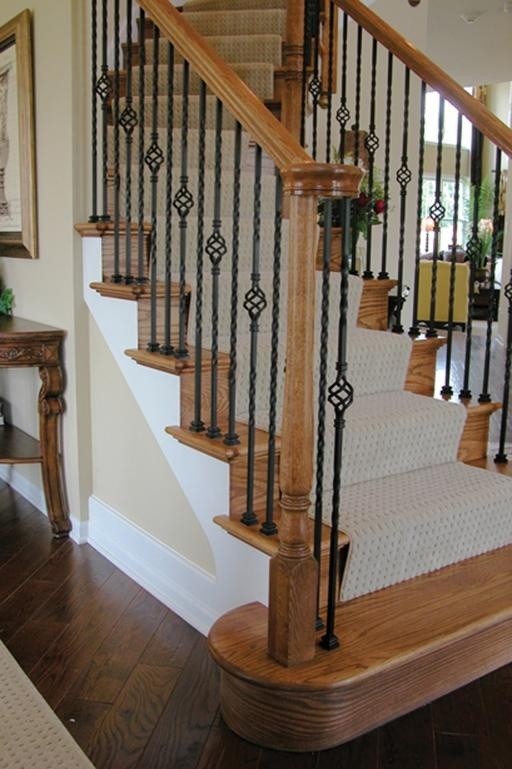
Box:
[316,146,389,236]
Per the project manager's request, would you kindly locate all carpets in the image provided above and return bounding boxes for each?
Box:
[1,639,100,769]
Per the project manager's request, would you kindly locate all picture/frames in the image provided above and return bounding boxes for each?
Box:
[0,6,38,261]
